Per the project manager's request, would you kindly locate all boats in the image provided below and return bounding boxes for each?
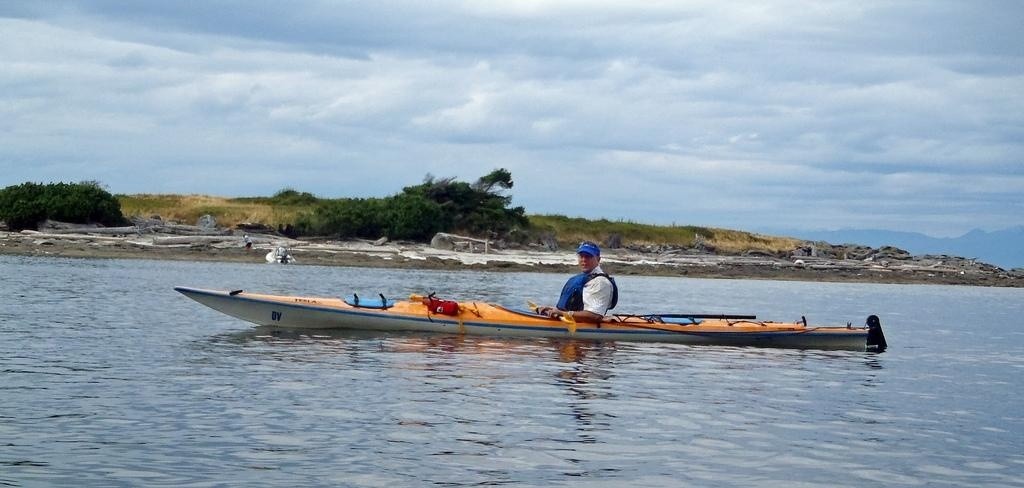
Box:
[172,283,890,358]
[264,246,296,264]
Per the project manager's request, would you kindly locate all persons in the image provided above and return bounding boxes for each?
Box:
[536,240,613,324]
[241,233,251,251]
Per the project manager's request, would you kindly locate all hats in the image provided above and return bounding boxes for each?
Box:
[576,241,599,257]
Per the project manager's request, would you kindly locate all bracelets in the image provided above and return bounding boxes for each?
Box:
[568,311,574,317]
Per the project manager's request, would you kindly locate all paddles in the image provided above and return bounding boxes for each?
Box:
[527,299,576,335]
